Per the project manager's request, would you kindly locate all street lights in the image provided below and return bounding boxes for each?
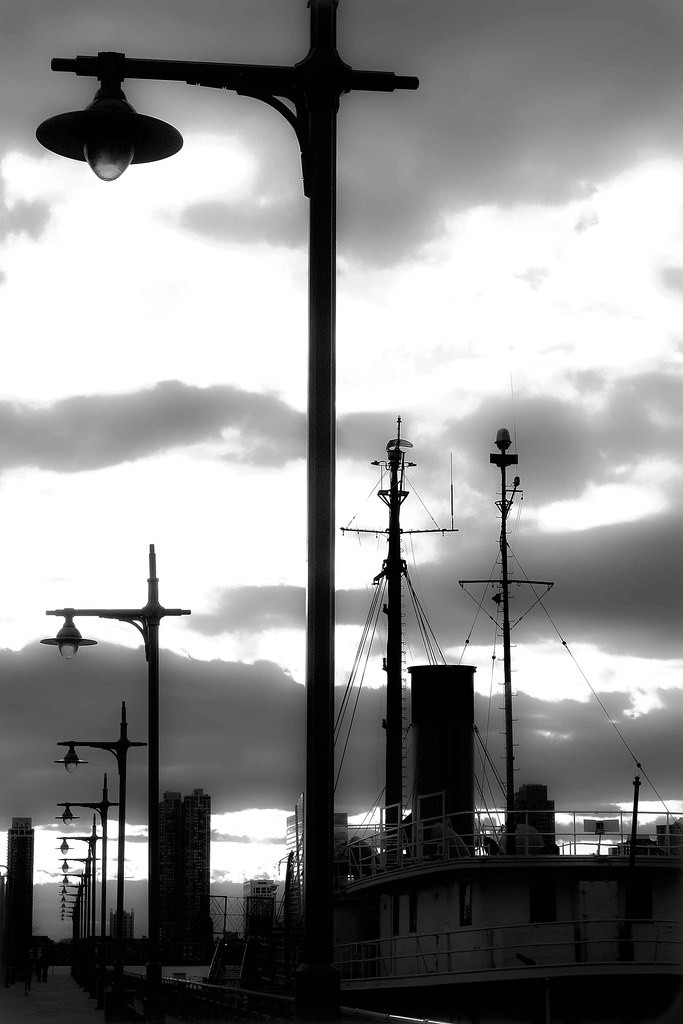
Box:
[38,542,195,1023]
[34,1,421,1023]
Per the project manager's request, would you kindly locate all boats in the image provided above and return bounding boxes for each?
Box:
[266,418,683,1004]
